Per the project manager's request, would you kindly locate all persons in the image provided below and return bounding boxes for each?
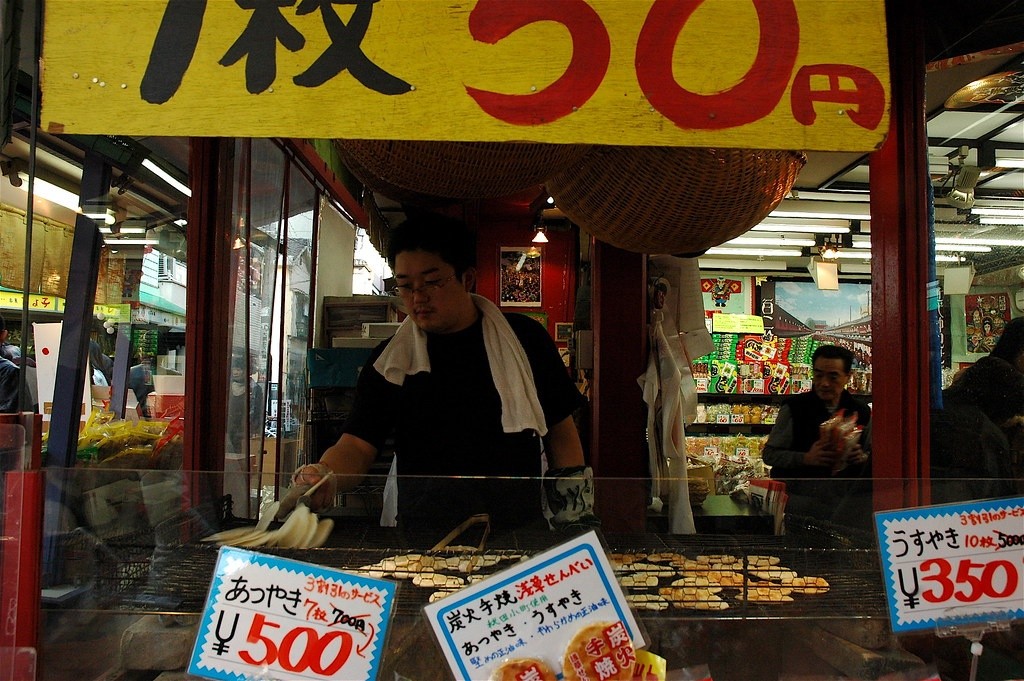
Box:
[90,329,265,453]
[761,344,880,535]
[292,214,593,532]
[0,314,34,414]
[942,316,1024,535]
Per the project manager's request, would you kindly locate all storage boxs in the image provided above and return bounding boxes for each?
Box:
[689,313,812,394]
[686,457,714,496]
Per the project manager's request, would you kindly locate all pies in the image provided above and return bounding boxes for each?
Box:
[345,547,831,611]
[486,659,558,681]
[560,624,635,681]
[199,501,333,549]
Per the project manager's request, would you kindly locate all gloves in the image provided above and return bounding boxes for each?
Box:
[543,466,602,536]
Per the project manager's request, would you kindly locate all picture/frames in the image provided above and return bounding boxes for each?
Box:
[496,243,544,309]
[555,322,574,342]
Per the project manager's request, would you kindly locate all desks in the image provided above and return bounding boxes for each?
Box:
[242,435,299,488]
[647,495,775,536]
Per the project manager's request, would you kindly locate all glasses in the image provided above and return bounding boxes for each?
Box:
[396,273,461,301]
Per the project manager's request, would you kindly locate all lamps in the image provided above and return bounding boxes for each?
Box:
[939,148,981,209]
[808,248,843,291]
[0,159,117,225]
[942,248,976,294]
[531,208,549,243]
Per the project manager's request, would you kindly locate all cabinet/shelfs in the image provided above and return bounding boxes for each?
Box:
[683,390,873,439]
